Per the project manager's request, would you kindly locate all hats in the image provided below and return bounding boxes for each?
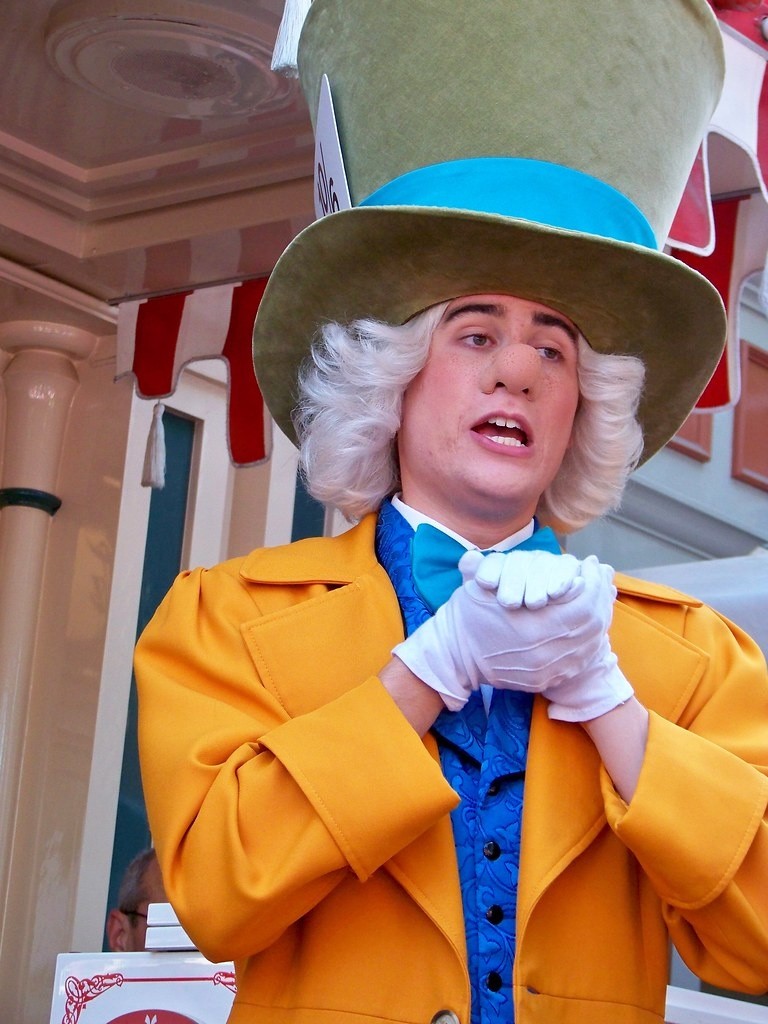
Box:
[251,154,730,474]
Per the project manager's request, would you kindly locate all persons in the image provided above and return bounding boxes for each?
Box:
[133,155,768,1024]
[106,847,168,951]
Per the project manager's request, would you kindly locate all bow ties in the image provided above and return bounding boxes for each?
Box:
[411,522,562,614]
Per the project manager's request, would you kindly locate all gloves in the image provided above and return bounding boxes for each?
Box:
[474,548,637,721]
[390,555,619,713]
